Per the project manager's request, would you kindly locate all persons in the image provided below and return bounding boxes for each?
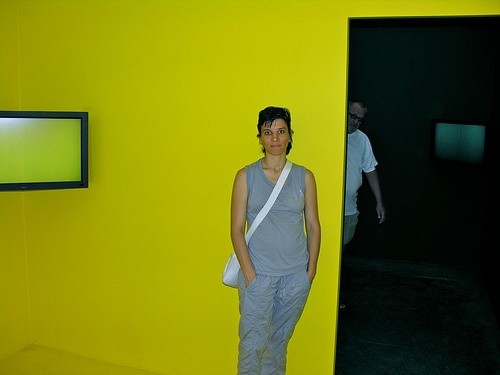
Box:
[230,107,323,375]
[339,98,387,309]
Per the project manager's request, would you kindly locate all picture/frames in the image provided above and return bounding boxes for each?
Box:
[0,111,88,191]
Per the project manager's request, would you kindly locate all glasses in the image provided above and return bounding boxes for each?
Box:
[349,111,366,122]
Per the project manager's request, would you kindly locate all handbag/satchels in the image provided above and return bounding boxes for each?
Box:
[222,251,241,288]
